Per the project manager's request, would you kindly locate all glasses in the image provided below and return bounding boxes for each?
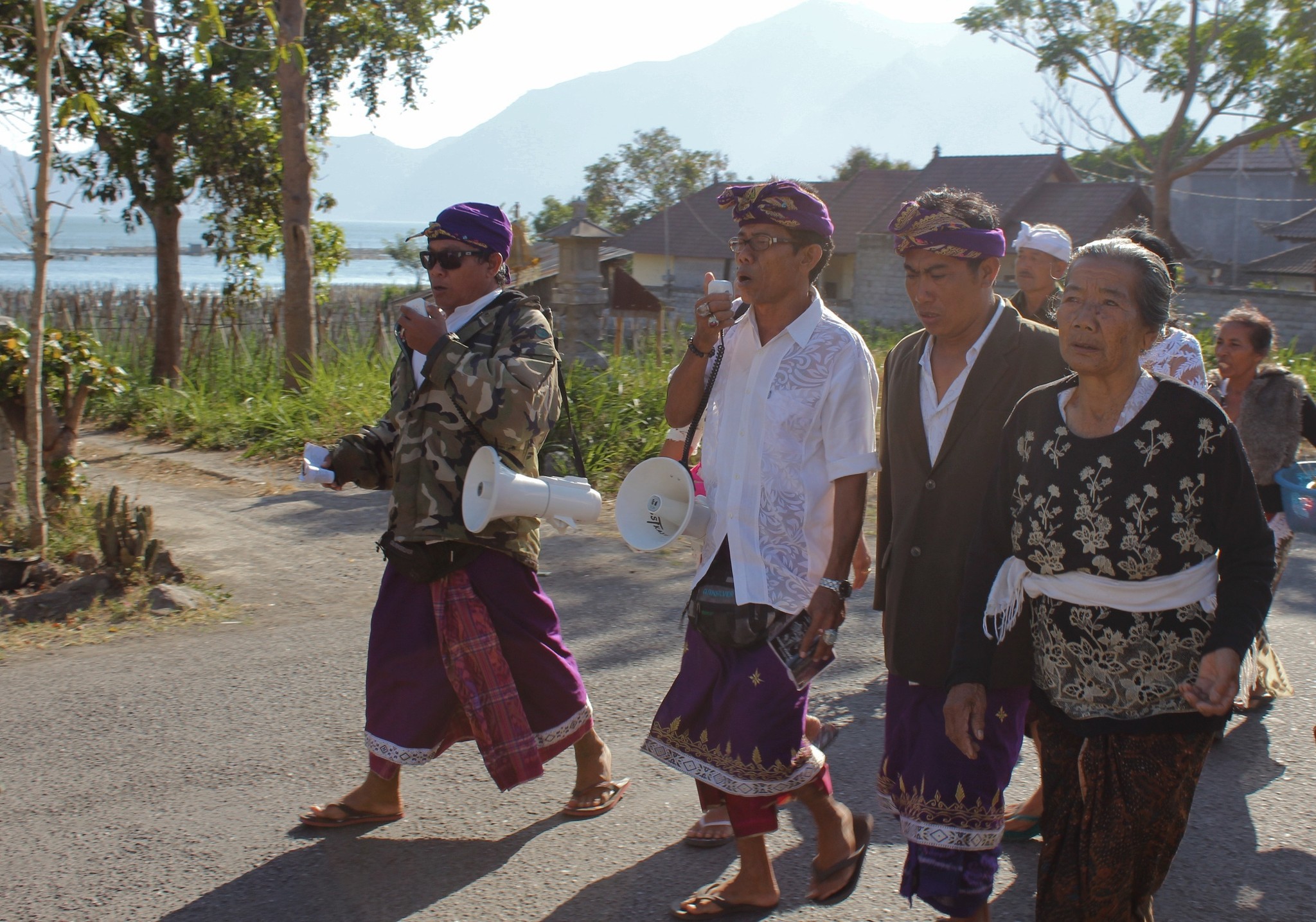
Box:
[728,233,816,254]
[420,250,494,269]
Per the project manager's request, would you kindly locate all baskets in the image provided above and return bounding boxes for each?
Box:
[1274,460,1316,535]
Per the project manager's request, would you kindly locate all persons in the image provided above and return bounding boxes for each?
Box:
[299,202,631,827]
[657,181,872,922]
[1011,223,1316,708]
[866,189,1078,922]
[946,239,1275,922]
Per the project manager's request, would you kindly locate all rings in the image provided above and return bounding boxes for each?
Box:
[708,315,718,328]
[819,629,837,646]
[400,328,406,338]
[696,303,712,316]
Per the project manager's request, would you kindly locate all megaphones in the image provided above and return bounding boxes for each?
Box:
[462,445,602,533]
[615,457,711,553]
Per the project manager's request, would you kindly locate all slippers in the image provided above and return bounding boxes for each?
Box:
[562,775,631,815]
[812,723,839,751]
[669,882,778,922]
[684,814,736,847]
[1003,801,1042,841]
[300,801,403,827]
[808,812,872,904]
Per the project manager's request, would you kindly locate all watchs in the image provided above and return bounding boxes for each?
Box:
[819,577,853,599]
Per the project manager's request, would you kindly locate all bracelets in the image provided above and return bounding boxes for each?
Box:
[687,335,716,358]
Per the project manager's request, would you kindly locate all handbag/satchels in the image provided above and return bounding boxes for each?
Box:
[374,530,484,583]
[693,575,784,651]
